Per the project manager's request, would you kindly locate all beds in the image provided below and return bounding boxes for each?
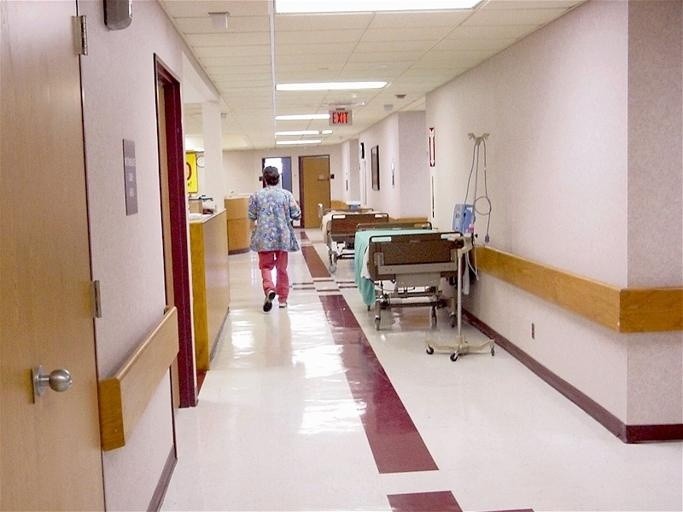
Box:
[354,221,463,331]
[322,208,388,273]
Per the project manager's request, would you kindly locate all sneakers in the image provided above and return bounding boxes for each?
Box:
[263,290,276,312]
[279,302,288,307]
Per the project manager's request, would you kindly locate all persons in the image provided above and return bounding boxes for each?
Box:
[246,165,303,313]
